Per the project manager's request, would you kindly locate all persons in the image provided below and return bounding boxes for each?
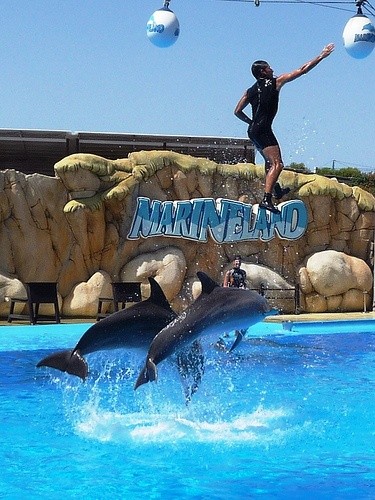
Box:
[233,43,335,215]
[222,255,246,337]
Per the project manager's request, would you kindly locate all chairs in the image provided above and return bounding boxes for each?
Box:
[6,280,61,325]
[96,280,143,321]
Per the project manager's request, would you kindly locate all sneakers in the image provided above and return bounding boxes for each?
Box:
[273,183,290,199]
[259,192,281,214]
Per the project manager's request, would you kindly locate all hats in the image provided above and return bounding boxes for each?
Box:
[230,256,240,262]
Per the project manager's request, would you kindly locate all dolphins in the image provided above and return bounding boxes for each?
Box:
[134,271,279,390]
[36,276,204,406]
